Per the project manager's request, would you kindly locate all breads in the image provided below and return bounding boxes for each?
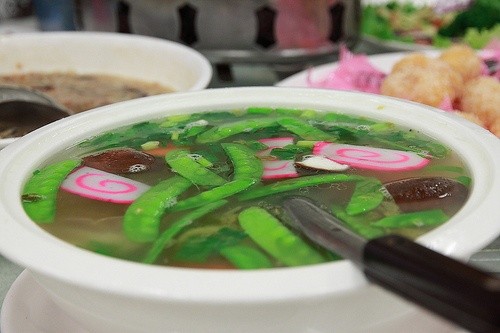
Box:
[379,42,500,136]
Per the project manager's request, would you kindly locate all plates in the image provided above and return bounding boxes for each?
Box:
[276,50,499,90]
[343,25,482,59]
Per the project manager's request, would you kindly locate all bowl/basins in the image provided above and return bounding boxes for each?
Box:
[0,86,500,333]
[0,30,214,146]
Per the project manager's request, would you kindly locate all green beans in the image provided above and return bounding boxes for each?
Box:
[21,113,448,271]
